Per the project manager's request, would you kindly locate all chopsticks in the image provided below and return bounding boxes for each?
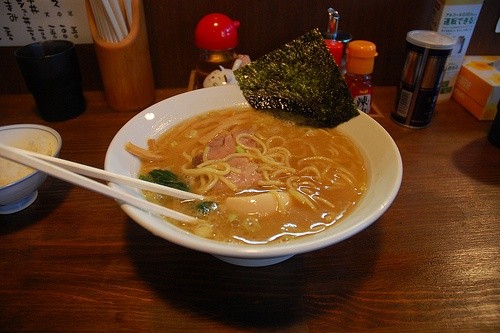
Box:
[0,142,205,224]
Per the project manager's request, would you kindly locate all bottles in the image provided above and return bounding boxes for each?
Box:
[323,40,343,72]
[345,39,379,117]
[195,12,240,89]
[389,28,456,129]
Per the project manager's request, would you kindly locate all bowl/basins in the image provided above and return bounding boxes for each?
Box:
[0,123,62,213]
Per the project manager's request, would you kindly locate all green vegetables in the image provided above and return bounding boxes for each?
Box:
[138,169,212,214]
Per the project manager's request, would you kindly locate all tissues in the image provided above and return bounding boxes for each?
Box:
[451,18,500,121]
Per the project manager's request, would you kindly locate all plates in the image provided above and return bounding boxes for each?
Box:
[103,85,405,265]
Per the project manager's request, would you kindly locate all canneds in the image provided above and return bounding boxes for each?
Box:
[390,30,455,128]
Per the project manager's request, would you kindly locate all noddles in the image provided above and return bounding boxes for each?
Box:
[140,104,368,245]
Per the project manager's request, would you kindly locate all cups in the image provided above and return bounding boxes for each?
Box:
[15,40,87,123]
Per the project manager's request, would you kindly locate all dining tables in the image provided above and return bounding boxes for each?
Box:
[0,85,500,333]
